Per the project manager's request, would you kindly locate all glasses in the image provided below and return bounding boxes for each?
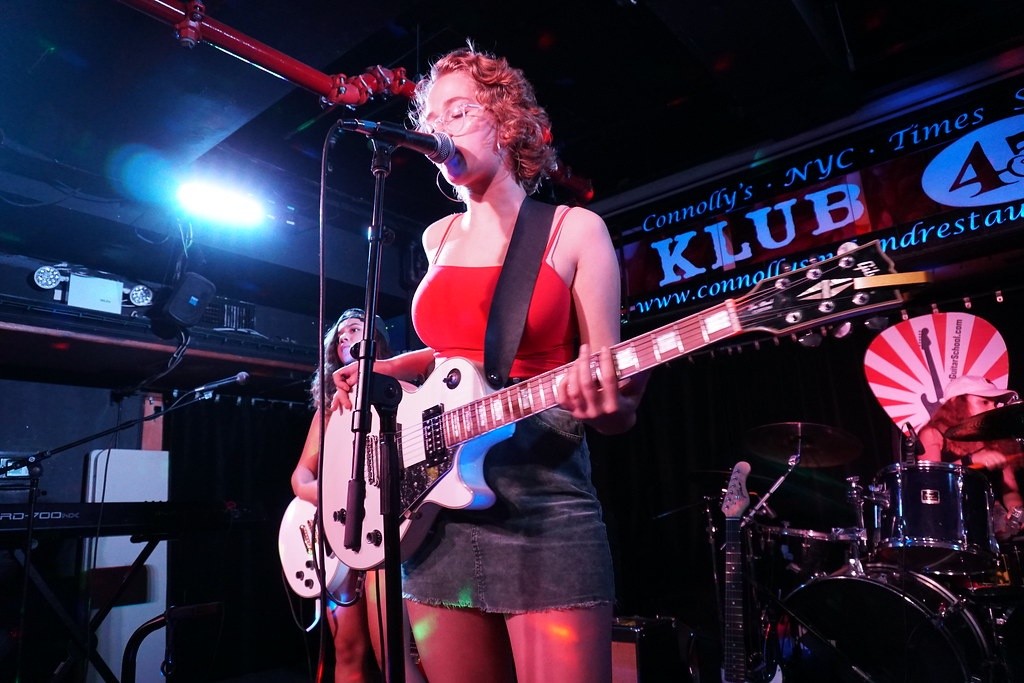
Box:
[420,102,484,136]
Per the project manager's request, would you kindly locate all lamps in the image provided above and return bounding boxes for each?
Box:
[33,266,155,315]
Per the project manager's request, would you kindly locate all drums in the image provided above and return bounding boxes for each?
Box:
[777,560,990,683]
[870,460,999,568]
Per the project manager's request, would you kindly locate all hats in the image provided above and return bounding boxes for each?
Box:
[943,375,1017,403]
[337,308,391,346]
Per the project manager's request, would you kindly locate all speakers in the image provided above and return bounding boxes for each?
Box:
[161,270,215,328]
[607,622,699,682]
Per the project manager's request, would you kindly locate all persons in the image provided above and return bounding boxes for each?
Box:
[331,50,631,683]
[917,376,1024,529]
[291,308,418,683]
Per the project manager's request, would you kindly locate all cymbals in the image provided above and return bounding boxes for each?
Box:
[742,421,864,466]
[944,402,1023,441]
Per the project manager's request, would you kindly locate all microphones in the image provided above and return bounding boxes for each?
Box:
[334,117,455,163]
[904,422,925,456]
[195,371,249,394]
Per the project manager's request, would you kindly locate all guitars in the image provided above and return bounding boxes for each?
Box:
[319,238,904,568]
[693,461,785,683]
[277,491,350,600]
